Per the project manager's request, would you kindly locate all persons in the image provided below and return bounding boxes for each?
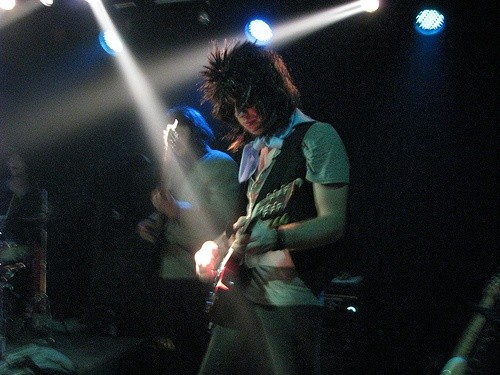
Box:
[0,152,54,332]
[197,42,351,375]
[135,106,247,351]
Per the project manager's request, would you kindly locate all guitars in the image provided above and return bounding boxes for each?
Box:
[135,116,178,257]
[204,180,305,325]
